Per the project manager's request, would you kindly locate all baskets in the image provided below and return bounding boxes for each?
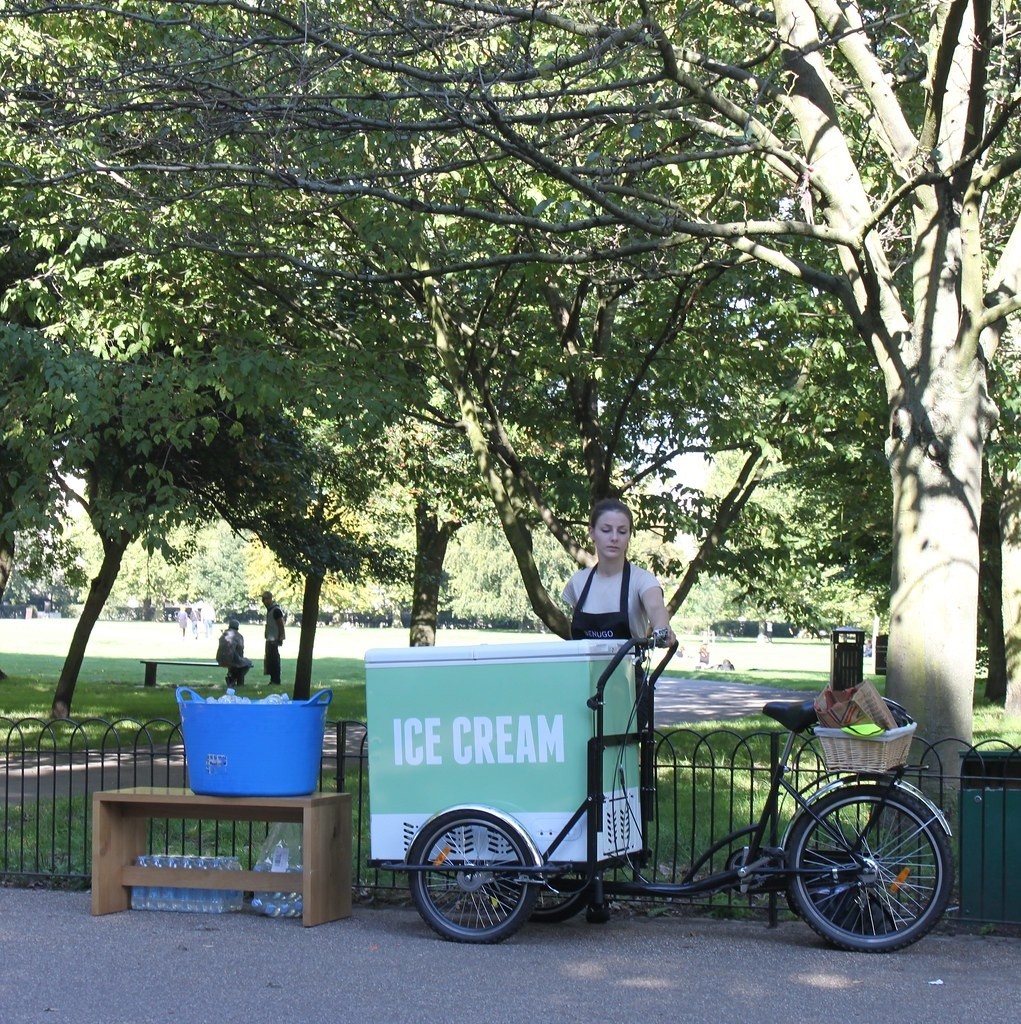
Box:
[813,721,916,773]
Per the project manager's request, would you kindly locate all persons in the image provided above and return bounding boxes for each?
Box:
[178,601,216,640]
[262,591,284,687]
[559,499,677,771]
[217,620,252,687]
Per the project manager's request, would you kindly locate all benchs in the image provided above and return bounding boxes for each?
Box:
[140,660,254,688]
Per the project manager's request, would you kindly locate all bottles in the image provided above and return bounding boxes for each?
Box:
[203,687,293,705]
[130,854,303,918]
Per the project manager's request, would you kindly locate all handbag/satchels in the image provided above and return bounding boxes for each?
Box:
[813,678,898,731]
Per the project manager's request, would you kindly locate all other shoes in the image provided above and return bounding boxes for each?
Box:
[225,677,232,687]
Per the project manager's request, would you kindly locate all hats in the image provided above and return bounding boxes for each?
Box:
[259,591,272,598]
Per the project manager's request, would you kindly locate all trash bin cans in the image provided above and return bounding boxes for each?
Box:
[956,749,1021,924]
[830,626,865,692]
[875,635,888,675]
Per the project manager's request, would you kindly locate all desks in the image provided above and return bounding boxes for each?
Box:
[92,787,353,928]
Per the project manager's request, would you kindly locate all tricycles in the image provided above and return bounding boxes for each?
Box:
[363,628,953,954]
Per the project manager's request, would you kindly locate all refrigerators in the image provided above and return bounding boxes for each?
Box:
[363,638,642,864]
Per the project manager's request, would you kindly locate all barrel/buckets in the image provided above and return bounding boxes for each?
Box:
[175,685,333,797]
